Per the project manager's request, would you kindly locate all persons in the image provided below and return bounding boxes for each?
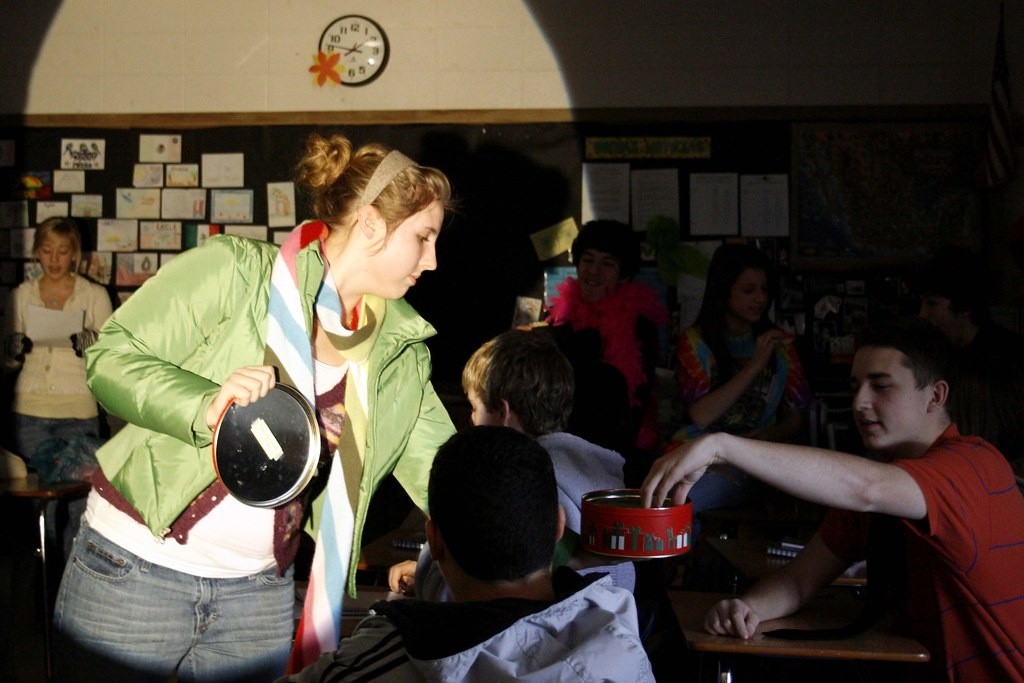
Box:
[282,220,1024,683]
[0,217,114,489]
[53,133,460,683]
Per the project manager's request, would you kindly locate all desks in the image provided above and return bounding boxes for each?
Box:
[1,475,91,683]
[665,589,930,683]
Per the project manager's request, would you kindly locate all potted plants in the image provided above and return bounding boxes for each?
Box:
[31,434,107,484]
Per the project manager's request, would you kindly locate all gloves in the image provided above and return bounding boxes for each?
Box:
[4,332,33,363]
[70,329,99,358]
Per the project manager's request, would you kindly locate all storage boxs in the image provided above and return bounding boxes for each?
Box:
[580,487,693,558]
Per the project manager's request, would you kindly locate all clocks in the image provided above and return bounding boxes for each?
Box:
[318,14,391,88]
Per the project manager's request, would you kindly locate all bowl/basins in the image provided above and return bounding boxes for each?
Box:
[581,488,692,559]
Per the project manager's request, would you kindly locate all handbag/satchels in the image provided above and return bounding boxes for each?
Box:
[28,429,109,487]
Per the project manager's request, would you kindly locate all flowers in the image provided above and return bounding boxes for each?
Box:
[308,51,345,89]
